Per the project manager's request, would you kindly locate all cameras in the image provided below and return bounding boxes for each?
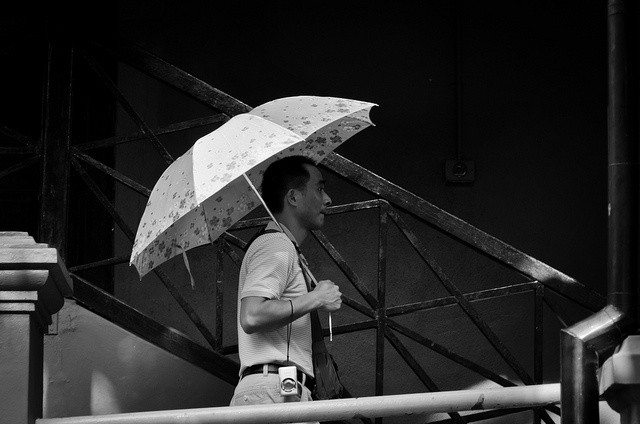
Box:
[278,366,297,397]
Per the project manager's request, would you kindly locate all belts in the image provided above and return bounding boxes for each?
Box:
[241,362,316,393]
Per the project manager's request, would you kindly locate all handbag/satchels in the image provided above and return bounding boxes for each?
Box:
[313,354,374,400]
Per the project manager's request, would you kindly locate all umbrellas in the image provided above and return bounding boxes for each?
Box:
[128,94,380,314]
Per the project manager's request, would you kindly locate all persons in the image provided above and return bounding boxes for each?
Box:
[229,155,343,423]
